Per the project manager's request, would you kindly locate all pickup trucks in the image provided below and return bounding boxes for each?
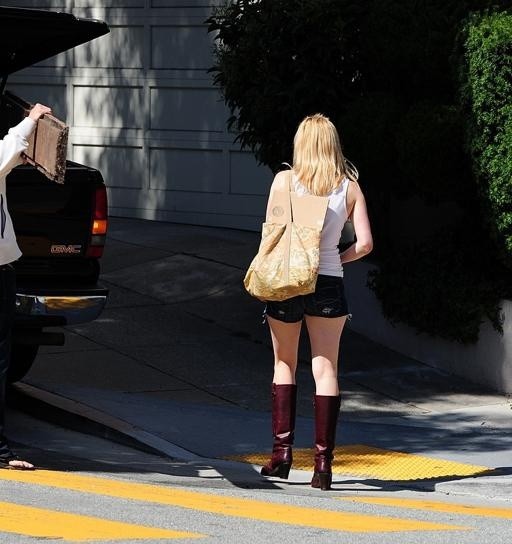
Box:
[0,160,110,385]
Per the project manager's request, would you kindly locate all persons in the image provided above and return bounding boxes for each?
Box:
[260,112,373,491]
[0,101,53,470]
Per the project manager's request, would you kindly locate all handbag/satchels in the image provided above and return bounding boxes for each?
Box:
[242,169,322,303]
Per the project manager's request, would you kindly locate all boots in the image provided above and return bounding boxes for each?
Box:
[311,393,341,490]
[260,383,298,479]
